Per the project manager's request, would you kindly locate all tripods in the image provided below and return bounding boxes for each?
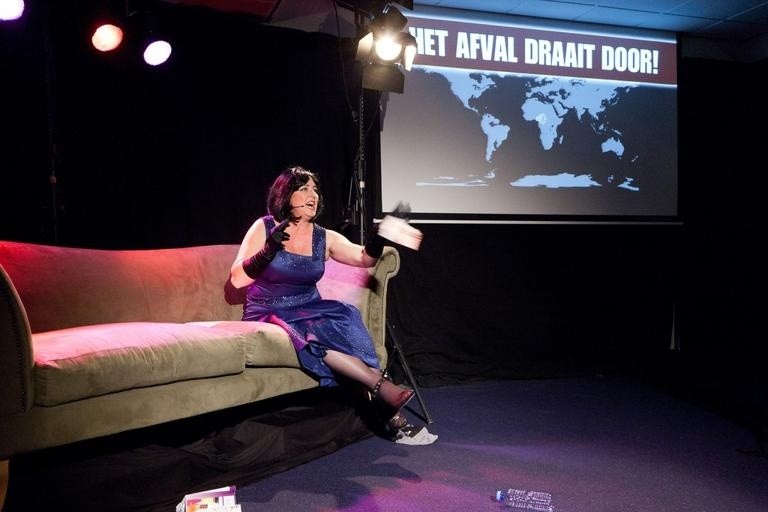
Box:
[358,86,435,426]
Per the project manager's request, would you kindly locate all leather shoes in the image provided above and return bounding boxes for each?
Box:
[374,378,415,411]
[389,411,408,428]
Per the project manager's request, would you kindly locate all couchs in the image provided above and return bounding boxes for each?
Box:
[0,233,403,512]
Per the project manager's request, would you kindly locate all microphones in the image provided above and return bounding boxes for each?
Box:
[290,205,304,208]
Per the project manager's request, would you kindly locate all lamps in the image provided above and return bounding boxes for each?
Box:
[342,3,439,435]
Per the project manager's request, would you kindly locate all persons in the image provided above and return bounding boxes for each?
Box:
[228,165,417,432]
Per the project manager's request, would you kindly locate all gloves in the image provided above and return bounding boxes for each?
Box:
[243,219,290,278]
[365,223,384,257]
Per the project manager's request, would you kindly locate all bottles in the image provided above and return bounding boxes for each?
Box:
[495,488,553,511]
